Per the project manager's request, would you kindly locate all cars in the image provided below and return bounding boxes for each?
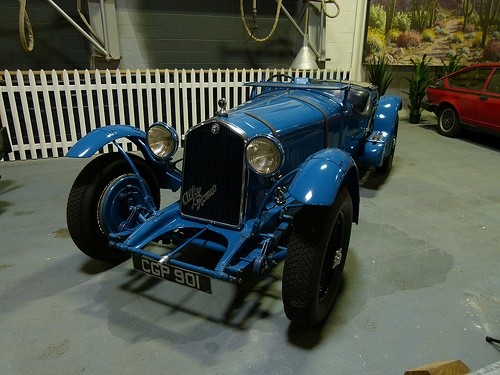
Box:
[65,75,403,329]
[419,64,500,137]
